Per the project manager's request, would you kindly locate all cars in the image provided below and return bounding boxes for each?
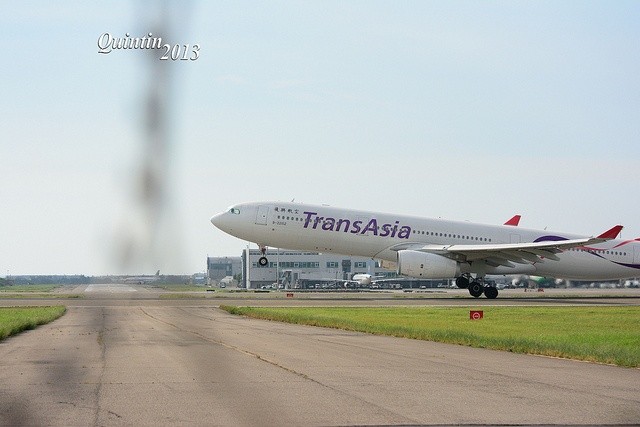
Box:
[259,282,334,289]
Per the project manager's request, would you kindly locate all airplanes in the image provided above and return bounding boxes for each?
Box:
[333,272,403,288]
[208,197,639,300]
